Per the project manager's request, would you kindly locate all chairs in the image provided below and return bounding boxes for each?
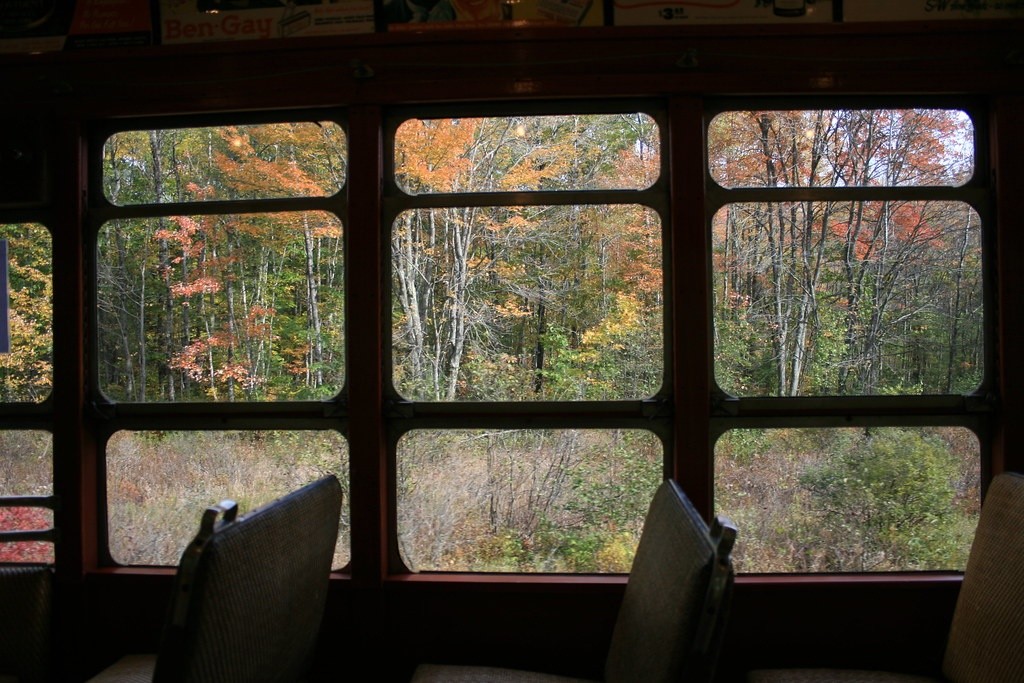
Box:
[749,470,1024,683]
[89,475,344,683]
[412,480,738,683]
